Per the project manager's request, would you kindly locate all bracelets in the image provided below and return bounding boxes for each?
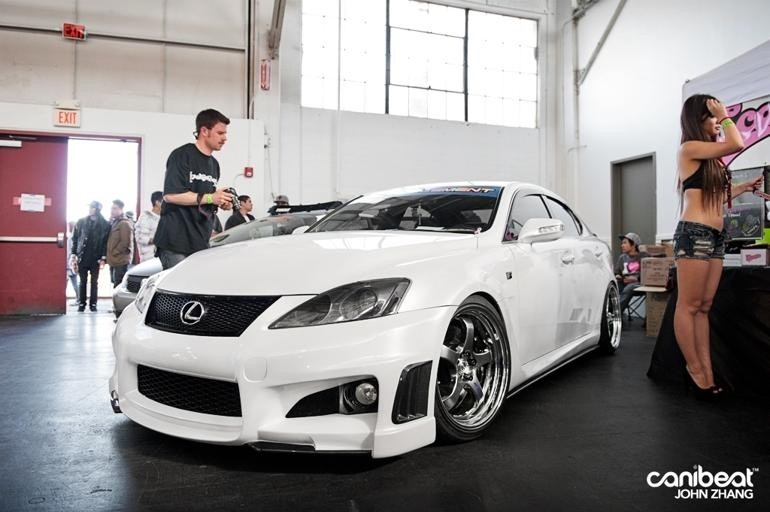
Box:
[720,121,734,129]
[719,116,732,124]
[207,193,213,205]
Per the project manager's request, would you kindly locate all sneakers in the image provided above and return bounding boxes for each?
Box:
[78,302,86,311]
[90,304,96,311]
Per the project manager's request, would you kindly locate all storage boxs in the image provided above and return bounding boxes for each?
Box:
[638,241,676,290]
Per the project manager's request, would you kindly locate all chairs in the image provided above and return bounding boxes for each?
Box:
[622,288,647,322]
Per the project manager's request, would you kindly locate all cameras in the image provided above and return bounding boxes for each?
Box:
[223,188,240,212]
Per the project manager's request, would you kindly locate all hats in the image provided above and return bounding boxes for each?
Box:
[86,201,102,210]
[273,195,289,203]
[618,232,642,246]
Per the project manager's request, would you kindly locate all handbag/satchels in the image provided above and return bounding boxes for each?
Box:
[132,240,141,266]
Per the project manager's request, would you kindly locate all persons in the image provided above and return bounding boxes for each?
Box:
[615,232,650,316]
[672,94,763,396]
[153,108,234,270]
[66,191,164,313]
[273,195,289,208]
[225,195,256,232]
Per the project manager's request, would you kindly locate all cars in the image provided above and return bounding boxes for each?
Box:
[106,181,622,460]
[113,201,406,322]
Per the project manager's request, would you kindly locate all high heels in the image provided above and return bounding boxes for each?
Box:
[682,367,724,403]
